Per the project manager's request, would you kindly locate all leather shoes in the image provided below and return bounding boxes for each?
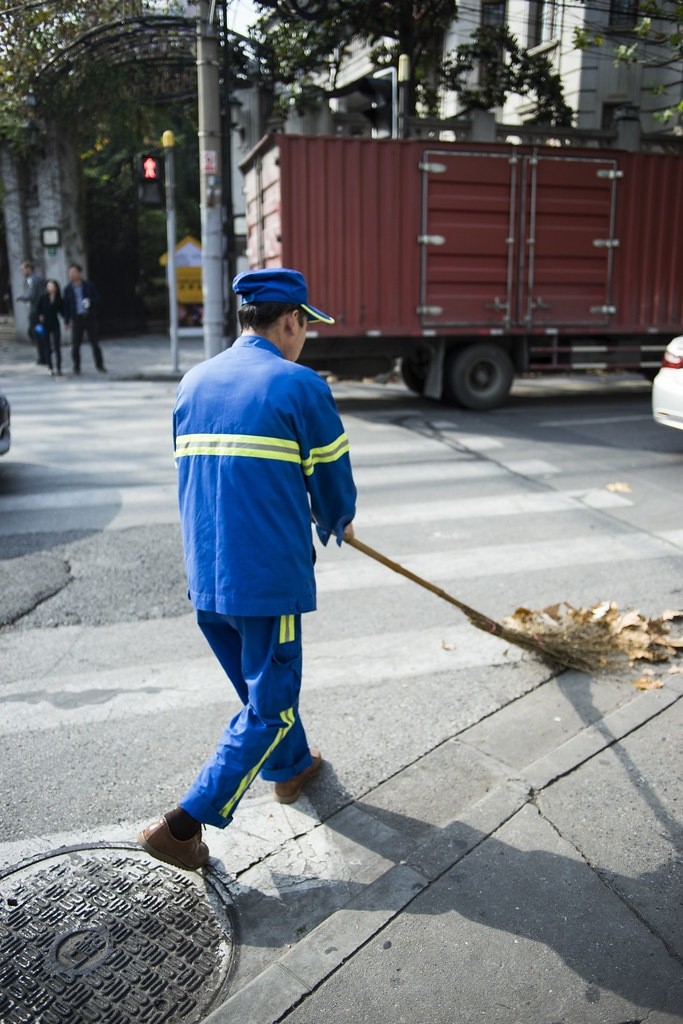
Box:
[138,815,209,872]
[273,748,321,803]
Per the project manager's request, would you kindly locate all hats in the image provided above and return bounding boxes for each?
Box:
[232,268,335,324]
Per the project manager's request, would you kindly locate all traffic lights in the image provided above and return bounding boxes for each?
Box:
[136,152,164,182]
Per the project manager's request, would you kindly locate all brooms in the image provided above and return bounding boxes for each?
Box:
[309,507,629,680]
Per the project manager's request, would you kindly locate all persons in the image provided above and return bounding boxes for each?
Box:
[15,259,50,364]
[137,270,357,870]
[63,263,106,373]
[34,279,65,376]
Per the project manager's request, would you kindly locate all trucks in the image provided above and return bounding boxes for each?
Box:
[237,130,683,411]
